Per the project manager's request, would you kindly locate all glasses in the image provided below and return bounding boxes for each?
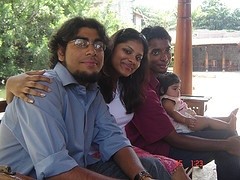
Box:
[67,37,107,51]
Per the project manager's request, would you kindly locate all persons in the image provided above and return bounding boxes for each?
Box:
[0,17,173,180]
[5,16,191,180]
[125,26,240,180]
[158,72,239,133]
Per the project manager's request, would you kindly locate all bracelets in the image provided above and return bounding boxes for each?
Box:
[134,171,152,180]
